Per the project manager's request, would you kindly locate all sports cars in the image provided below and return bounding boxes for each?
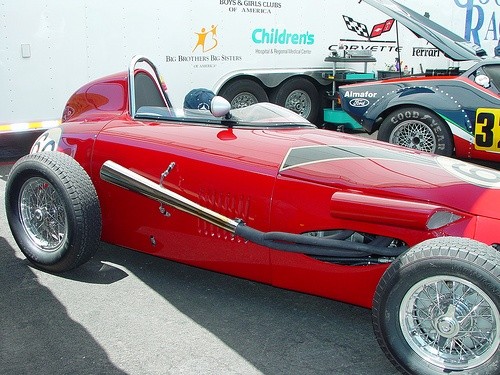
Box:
[338,60,499,162]
[4,55,500,374]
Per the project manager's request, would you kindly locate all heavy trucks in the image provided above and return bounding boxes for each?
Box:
[1,0,498,141]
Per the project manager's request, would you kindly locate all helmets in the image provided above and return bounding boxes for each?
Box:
[183,88,216,116]
